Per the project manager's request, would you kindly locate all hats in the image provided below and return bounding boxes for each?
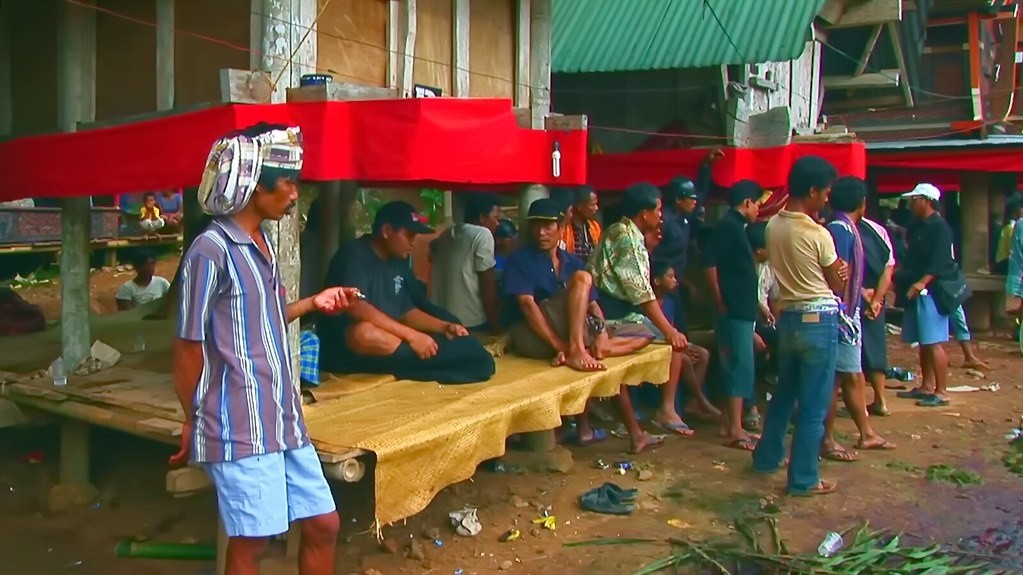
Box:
[901,182,941,201]
[376,200,436,233]
[527,199,561,220]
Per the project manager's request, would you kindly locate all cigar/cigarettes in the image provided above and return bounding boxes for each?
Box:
[354,291,365,299]
[771,324,777,330]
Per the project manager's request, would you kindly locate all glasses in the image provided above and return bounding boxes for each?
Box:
[912,196,927,199]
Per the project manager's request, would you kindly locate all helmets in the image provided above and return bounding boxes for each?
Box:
[669,177,699,199]
[494,218,517,239]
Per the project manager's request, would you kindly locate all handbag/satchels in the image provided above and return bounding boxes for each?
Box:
[928,268,971,315]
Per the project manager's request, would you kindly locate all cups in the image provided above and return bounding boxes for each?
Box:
[818,532,844,557]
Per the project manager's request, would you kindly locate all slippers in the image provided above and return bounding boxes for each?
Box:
[853,440,898,450]
[866,403,892,416]
[820,445,859,461]
[577,428,609,445]
[722,439,757,451]
[579,489,635,514]
[836,407,851,418]
[808,479,836,494]
[896,387,926,398]
[916,394,950,406]
[585,482,638,501]
[651,419,696,438]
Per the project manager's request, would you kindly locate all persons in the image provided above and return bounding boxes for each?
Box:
[749,155,849,497]
[114,147,1023,461]
[170,122,364,575]
